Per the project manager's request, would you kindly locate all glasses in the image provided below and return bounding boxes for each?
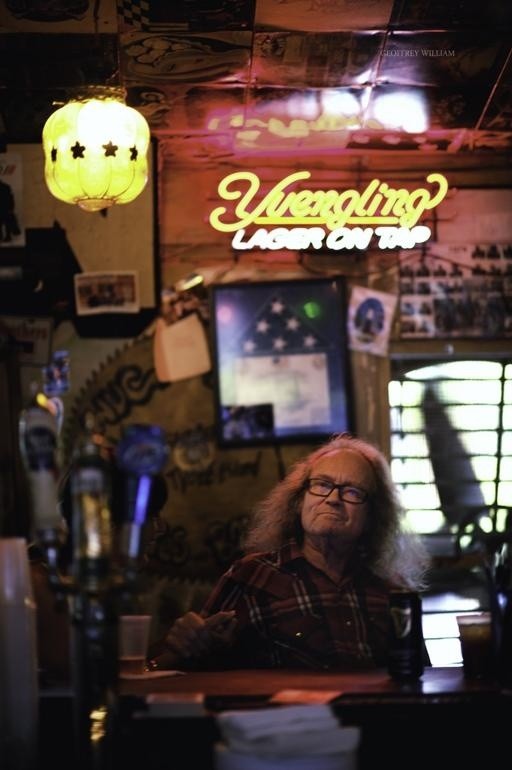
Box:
[301,478,367,505]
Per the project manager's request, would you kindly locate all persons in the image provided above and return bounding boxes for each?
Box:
[166,431,435,675]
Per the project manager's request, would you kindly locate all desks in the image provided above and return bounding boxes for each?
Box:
[120,660,511,767]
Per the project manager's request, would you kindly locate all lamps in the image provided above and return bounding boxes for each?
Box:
[42,2,151,212]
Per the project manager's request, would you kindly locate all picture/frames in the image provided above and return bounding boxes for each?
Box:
[204,275,359,450]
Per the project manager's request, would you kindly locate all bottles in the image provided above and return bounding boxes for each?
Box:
[20,451,131,748]
